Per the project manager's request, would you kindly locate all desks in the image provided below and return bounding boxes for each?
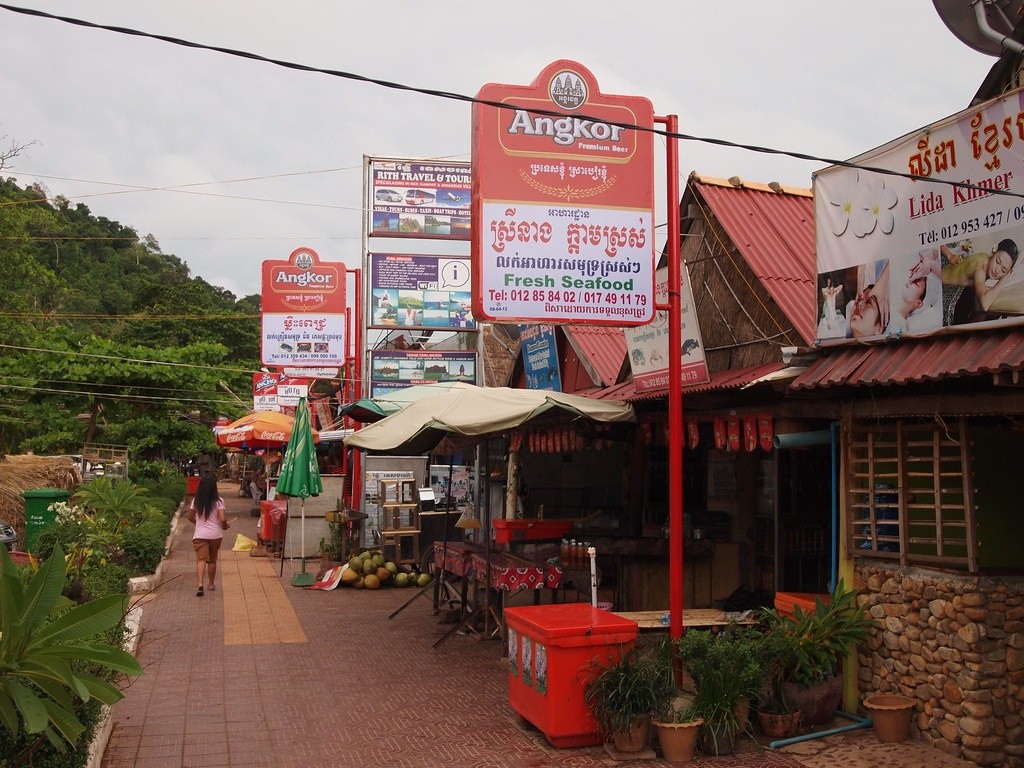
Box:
[609,608,761,632]
[472,551,564,641]
[417,511,464,563]
[784,549,831,593]
[432,540,502,620]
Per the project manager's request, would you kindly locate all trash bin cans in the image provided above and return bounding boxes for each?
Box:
[186,476,202,494]
[0,521,18,551]
[18,488,76,553]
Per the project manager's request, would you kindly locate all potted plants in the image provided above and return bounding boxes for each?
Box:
[650,706,705,762]
[759,670,803,740]
[673,610,772,735]
[695,669,764,760]
[319,498,352,572]
[583,627,676,753]
[758,576,881,726]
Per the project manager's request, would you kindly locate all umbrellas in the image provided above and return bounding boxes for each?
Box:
[276,398,324,577]
[335,381,637,638]
[215,411,320,502]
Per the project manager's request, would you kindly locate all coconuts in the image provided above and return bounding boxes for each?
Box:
[339,551,431,589]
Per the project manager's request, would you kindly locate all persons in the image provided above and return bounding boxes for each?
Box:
[188,473,230,597]
[890,247,940,333]
[940,239,1019,312]
[821,259,891,337]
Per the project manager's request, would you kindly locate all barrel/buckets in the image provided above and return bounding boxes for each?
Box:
[186,477,201,495]
[863,694,916,743]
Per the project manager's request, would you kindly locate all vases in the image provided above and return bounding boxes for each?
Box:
[862,695,916,743]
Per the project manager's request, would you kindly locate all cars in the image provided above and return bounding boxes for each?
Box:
[47,455,103,477]
[0,519,18,551]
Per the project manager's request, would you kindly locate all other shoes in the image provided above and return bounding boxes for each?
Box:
[196,585,204,597]
[208,586,215,590]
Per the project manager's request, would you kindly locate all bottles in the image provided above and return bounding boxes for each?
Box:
[560,539,590,570]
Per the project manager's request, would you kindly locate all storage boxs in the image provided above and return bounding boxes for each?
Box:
[501,603,640,750]
[258,499,288,542]
[492,517,575,544]
[184,476,201,495]
[774,592,831,625]
